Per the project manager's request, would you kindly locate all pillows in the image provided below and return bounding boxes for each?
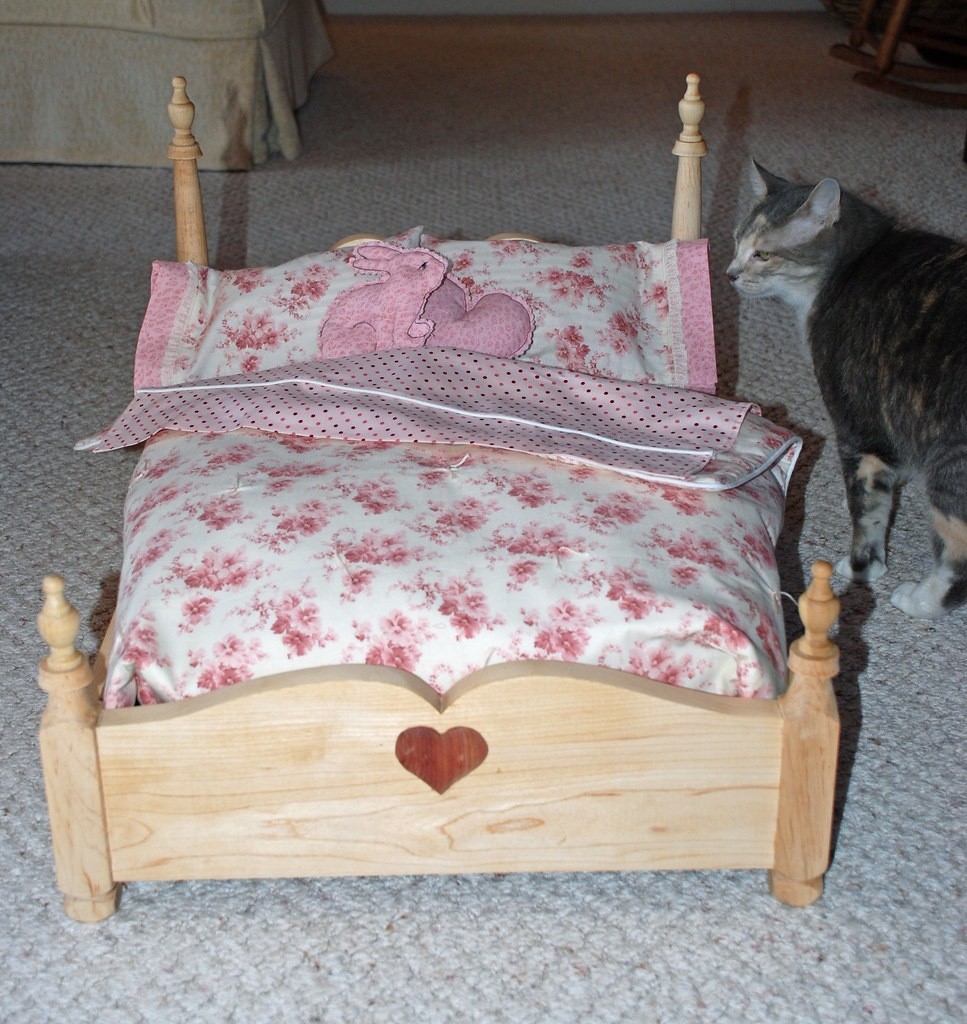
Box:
[132,225,717,393]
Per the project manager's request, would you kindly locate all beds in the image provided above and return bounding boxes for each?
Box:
[36,74,840,921]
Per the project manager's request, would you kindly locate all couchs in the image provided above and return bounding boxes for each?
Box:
[0,0,334,168]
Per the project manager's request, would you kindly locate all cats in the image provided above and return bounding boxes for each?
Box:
[724,157,967,618]
